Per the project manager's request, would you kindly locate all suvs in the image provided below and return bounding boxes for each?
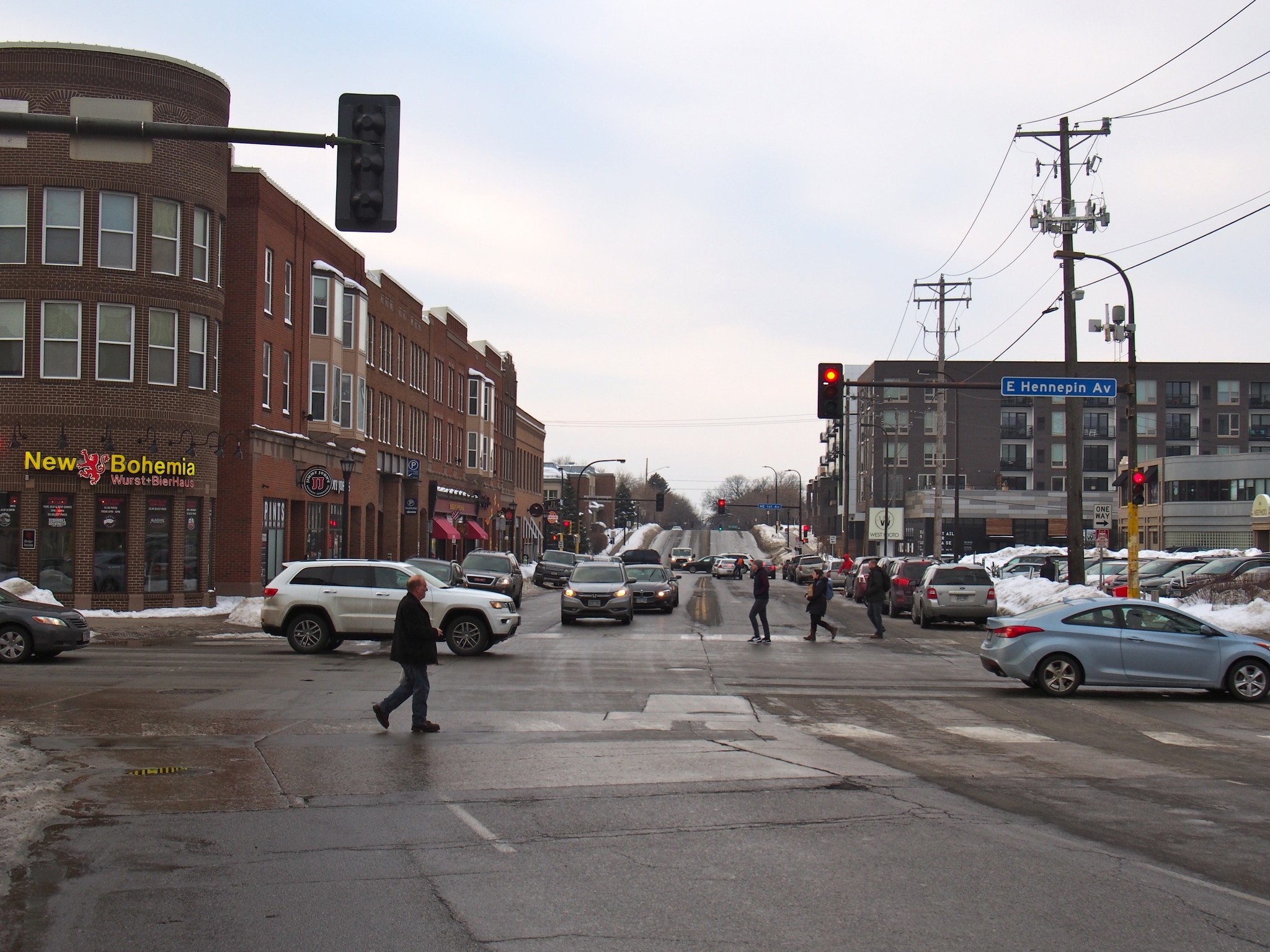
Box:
[559,561,637,625]
[404,554,468,588]
[532,549,577,588]
[260,558,521,657]
[459,547,524,609]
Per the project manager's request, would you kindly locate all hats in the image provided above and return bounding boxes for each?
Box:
[754,559,763,568]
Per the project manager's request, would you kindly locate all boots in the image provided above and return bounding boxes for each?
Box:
[826,623,838,639]
[803,628,817,641]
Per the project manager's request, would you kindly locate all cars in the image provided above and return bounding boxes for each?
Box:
[671,526,682,531]
[0,588,91,665]
[624,563,682,613]
[575,547,777,581]
[979,595,1270,703]
[780,543,1270,630]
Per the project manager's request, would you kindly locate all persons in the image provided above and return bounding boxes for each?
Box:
[748,559,772,644]
[369,574,443,732]
[836,553,855,595]
[732,557,744,579]
[865,559,886,638]
[803,568,838,641]
[1040,556,1060,582]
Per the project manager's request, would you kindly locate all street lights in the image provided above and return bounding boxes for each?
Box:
[576,459,626,555]
[762,465,802,556]
[557,467,564,508]
[1053,250,1142,597]
[509,499,518,554]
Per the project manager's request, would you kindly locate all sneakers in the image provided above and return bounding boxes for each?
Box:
[411,720,440,732]
[747,636,762,643]
[373,703,389,729]
[757,638,771,644]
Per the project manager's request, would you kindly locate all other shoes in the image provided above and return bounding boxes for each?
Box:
[875,626,886,635]
[840,592,845,595]
[870,634,883,638]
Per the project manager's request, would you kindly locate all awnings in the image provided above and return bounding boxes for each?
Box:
[465,521,489,539]
[432,517,461,540]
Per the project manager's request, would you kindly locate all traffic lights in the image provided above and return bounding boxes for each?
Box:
[803,526,809,544]
[718,500,725,515]
[656,494,664,511]
[552,520,583,542]
[818,363,844,419]
[1130,472,1144,508]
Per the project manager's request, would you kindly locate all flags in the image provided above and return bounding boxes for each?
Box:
[451,509,460,521]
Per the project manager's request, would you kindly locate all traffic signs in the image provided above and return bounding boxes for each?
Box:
[405,498,418,515]
[1093,504,1112,529]
[407,458,420,479]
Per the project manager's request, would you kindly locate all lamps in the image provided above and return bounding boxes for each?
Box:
[56,421,68,447]
[100,424,115,452]
[9,421,28,449]
[137,426,159,452]
[168,429,243,460]
[302,411,313,421]
[497,511,505,519]
[455,457,462,463]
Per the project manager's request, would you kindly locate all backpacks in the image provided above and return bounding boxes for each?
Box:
[737,558,743,566]
[881,569,891,592]
[816,581,833,600]
